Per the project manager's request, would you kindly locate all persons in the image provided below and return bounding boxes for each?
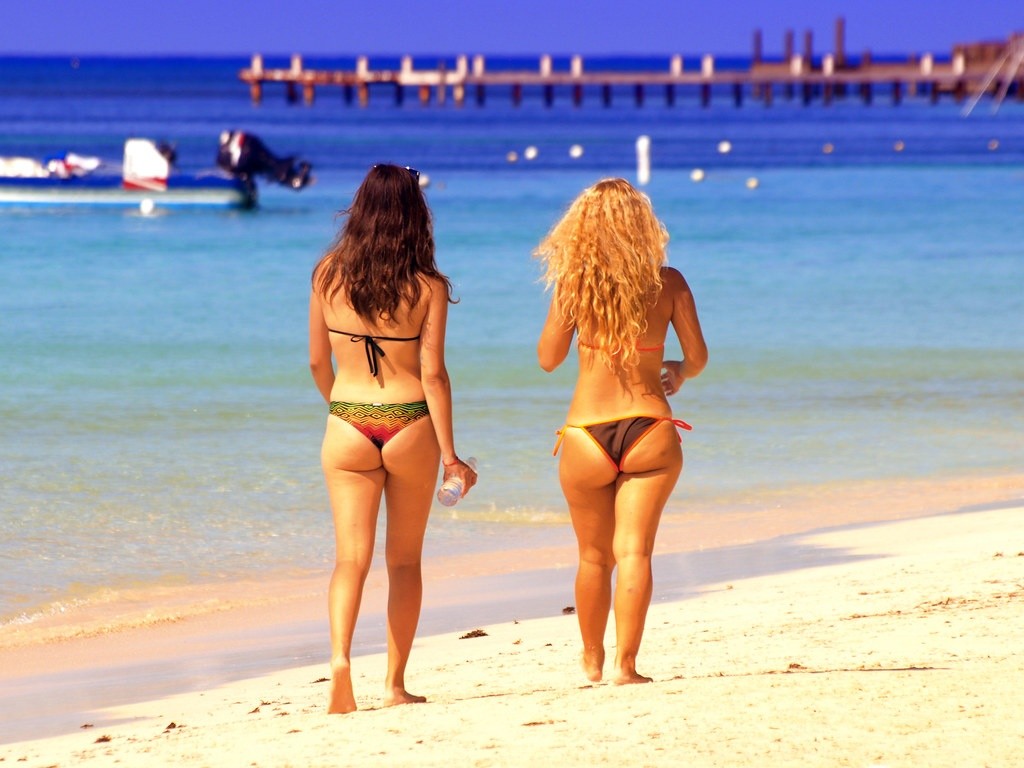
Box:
[531,177,709,687]
[309,164,479,715]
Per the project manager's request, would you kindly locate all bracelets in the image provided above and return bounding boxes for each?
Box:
[442,459,460,467]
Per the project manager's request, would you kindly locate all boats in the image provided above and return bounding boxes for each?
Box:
[0,130,314,207]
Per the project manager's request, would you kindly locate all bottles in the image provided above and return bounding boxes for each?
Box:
[437,457,478,506]
